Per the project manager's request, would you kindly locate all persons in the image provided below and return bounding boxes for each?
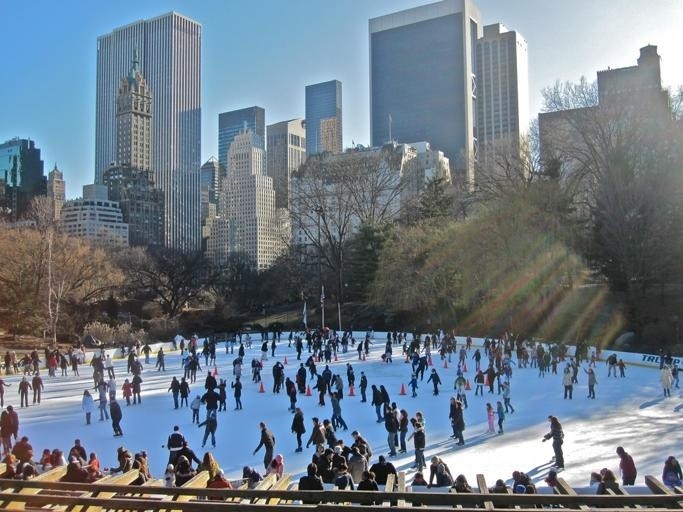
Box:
[0,322,682,505]
